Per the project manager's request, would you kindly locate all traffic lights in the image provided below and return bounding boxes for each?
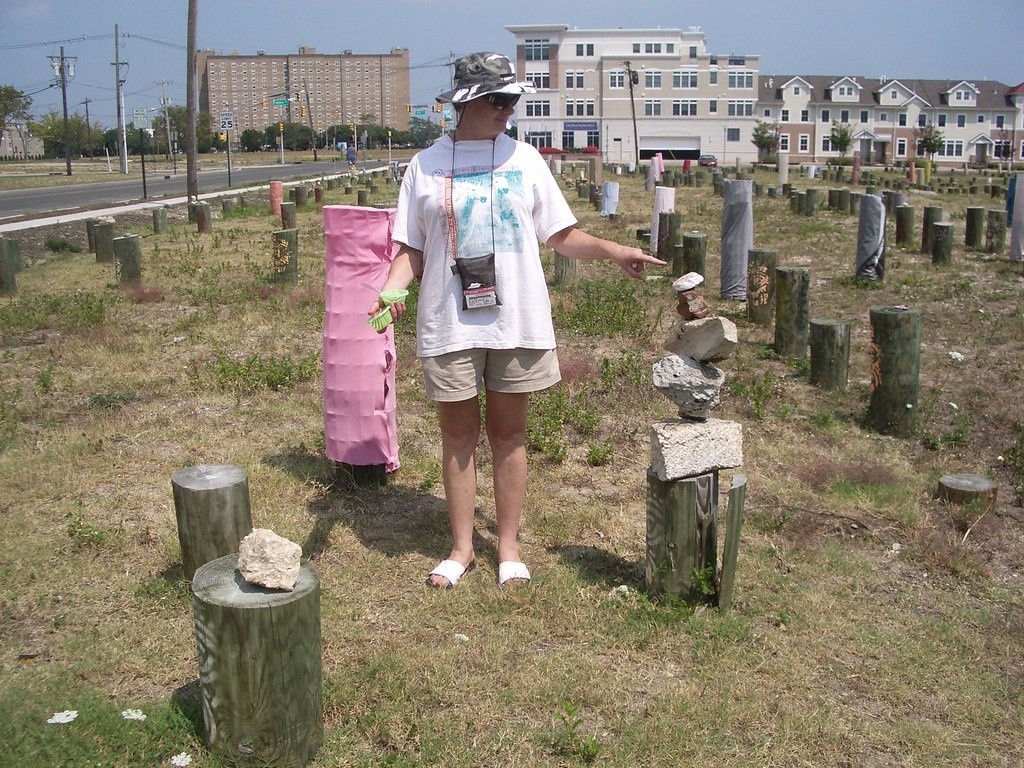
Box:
[301,106,307,118]
[436,103,443,113]
[407,104,413,113]
[294,91,301,103]
[262,96,268,109]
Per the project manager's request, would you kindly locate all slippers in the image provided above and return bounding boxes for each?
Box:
[425,558,477,589]
[497,560,531,589]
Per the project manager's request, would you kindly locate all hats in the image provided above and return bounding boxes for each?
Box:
[435,53,539,105]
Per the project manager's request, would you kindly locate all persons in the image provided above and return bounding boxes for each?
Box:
[346,143,358,170]
[365,52,669,595]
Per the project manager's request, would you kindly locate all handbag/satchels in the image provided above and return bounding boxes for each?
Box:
[457,253,504,311]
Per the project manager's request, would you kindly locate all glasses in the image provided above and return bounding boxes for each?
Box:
[480,93,523,109]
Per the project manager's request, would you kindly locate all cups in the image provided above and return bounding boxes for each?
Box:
[380,290,409,307]
[367,306,394,331]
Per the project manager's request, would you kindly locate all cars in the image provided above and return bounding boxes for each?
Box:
[697,154,718,167]
